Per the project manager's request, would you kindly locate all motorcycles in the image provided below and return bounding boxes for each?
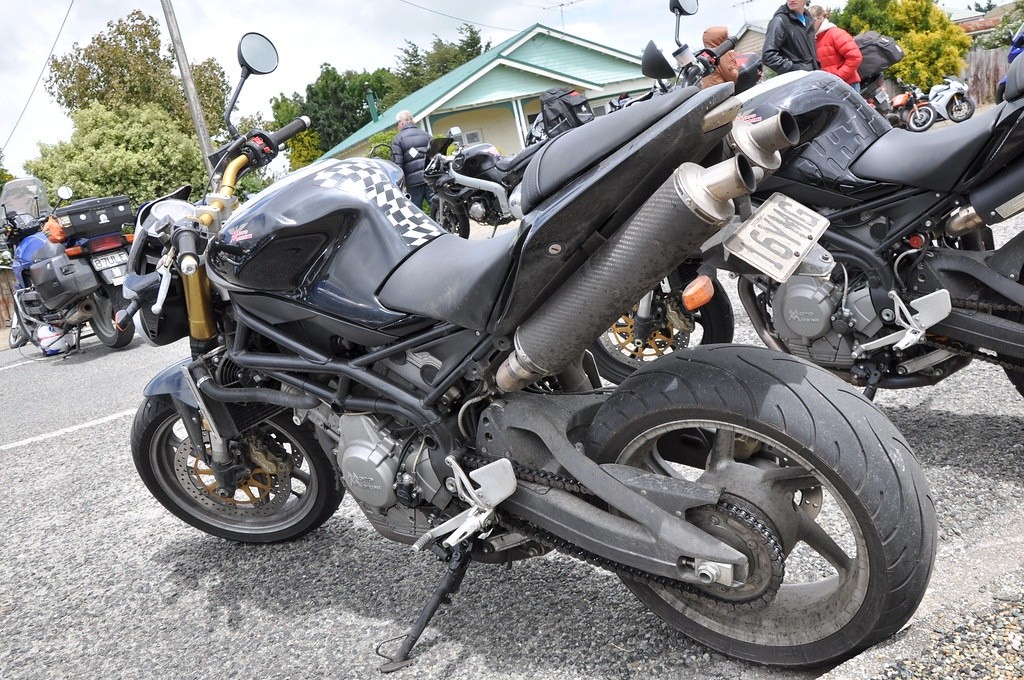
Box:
[924,74,975,123]
[1,178,138,358]
[523,0,1024,399]
[525,75,655,149]
[408,124,549,240]
[888,77,937,133]
[122,31,942,673]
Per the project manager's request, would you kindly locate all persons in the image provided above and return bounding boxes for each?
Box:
[809,6,862,94]
[762,0,818,74]
[702,25,739,87]
[392,111,432,209]
